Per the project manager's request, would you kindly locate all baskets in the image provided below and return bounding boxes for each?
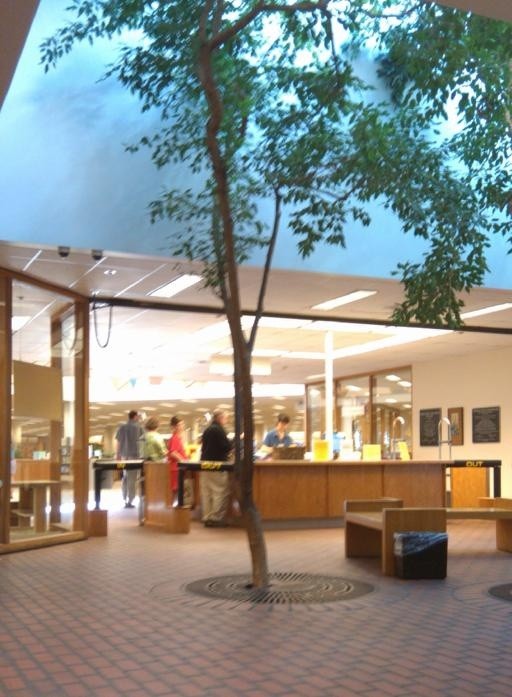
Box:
[272,446,308,460]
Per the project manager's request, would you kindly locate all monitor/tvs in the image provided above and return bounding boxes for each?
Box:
[272,446,305,460]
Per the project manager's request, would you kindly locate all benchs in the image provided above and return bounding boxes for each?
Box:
[341,496,512,575]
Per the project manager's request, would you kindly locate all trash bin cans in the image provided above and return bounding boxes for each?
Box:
[394,531,448,579]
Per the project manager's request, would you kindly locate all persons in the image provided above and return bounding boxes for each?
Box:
[135,414,166,460]
[113,407,143,508]
[258,412,297,459]
[168,415,193,508]
[198,408,233,528]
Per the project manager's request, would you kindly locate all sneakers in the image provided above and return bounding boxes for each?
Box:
[205,520,226,529]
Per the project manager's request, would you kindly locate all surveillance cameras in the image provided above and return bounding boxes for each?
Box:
[91,249,103,261]
[58,248,70,257]
